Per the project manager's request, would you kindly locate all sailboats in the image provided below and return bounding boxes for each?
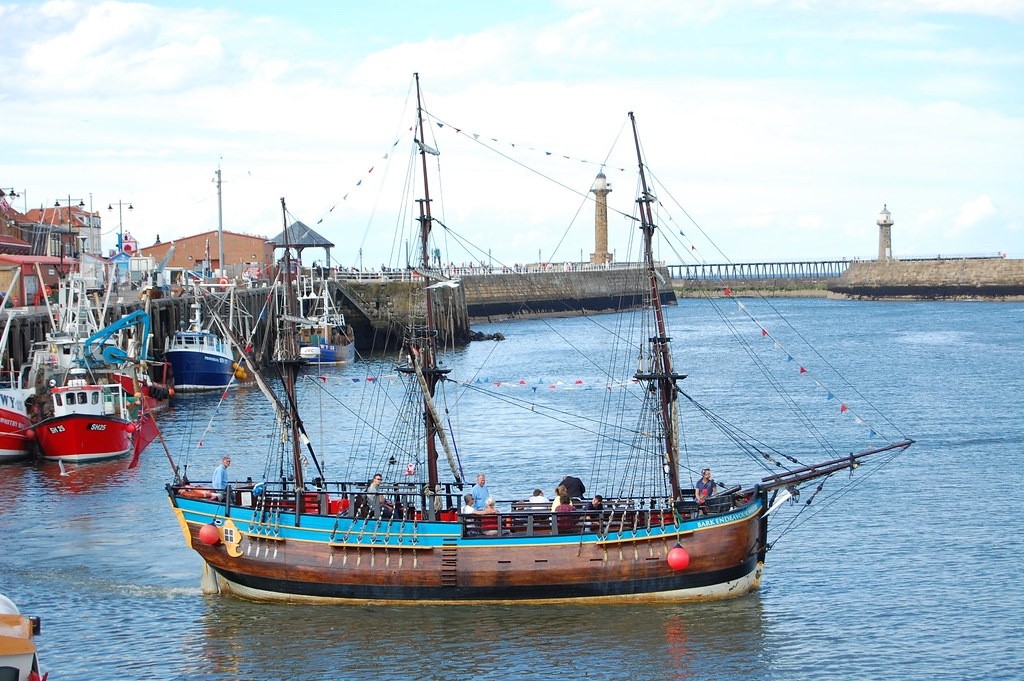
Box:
[1,166,357,462]
[165,73,917,605]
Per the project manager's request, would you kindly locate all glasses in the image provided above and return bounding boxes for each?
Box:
[375,478,382,480]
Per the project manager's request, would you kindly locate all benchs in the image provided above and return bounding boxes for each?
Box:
[263,492,321,516]
[510,501,635,526]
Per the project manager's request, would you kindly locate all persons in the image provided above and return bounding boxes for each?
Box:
[480,496,511,537]
[555,475,586,520]
[366,473,400,520]
[212,455,231,503]
[469,473,491,533]
[554,493,581,534]
[690,468,718,520]
[454,493,501,536]
[112,271,171,300]
[583,494,604,534]
[512,500,528,526]
[333,259,574,280]
[548,485,573,530]
[527,489,552,526]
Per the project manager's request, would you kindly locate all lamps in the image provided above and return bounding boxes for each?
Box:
[9,191,16,200]
[129,205,134,212]
[54,202,61,210]
[108,206,113,212]
[0,189,5,201]
[16,194,20,199]
[79,202,85,209]
